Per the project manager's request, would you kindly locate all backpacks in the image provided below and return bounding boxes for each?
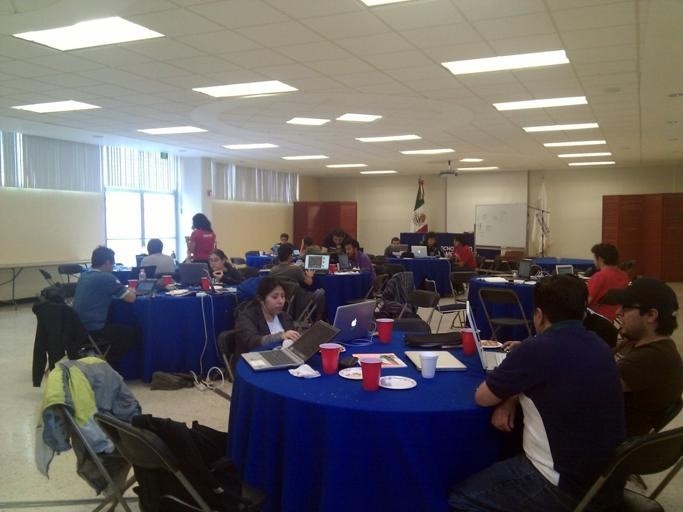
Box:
[133,414,252,511]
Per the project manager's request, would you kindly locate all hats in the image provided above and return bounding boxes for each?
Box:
[598,278,678,310]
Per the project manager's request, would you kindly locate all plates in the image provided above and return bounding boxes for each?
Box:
[481,338,503,349]
[336,365,417,392]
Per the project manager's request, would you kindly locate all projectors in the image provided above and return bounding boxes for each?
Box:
[439,171,458,177]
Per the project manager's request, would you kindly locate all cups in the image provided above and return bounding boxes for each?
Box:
[375,318,394,344]
[318,342,341,376]
[329,264,337,274]
[358,356,383,394]
[418,351,438,380]
[127,274,209,292]
[459,328,480,356]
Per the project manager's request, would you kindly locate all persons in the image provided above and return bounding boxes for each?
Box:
[594,278,682,476]
[184,213,216,264]
[268,245,326,324]
[589,243,629,319]
[233,278,302,368]
[271,229,476,294]
[139,238,176,275]
[500,275,618,351]
[207,249,241,284]
[72,245,139,365]
[446,274,624,510]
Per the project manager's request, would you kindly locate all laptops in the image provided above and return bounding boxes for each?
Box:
[131,255,156,297]
[304,253,351,276]
[556,265,574,275]
[464,301,507,374]
[292,248,300,257]
[240,320,342,372]
[518,258,533,278]
[330,300,376,343]
[389,244,428,257]
[179,263,210,286]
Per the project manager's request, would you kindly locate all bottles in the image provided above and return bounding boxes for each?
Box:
[137,268,146,280]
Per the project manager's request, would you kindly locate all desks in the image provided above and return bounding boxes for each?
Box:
[0,260,92,312]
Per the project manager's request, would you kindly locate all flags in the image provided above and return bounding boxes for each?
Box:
[412,183,428,233]
[529,183,553,256]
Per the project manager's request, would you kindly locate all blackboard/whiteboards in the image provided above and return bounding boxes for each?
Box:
[473,204,527,252]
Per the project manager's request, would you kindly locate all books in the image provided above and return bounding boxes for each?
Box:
[404,349,468,372]
[352,351,409,372]
